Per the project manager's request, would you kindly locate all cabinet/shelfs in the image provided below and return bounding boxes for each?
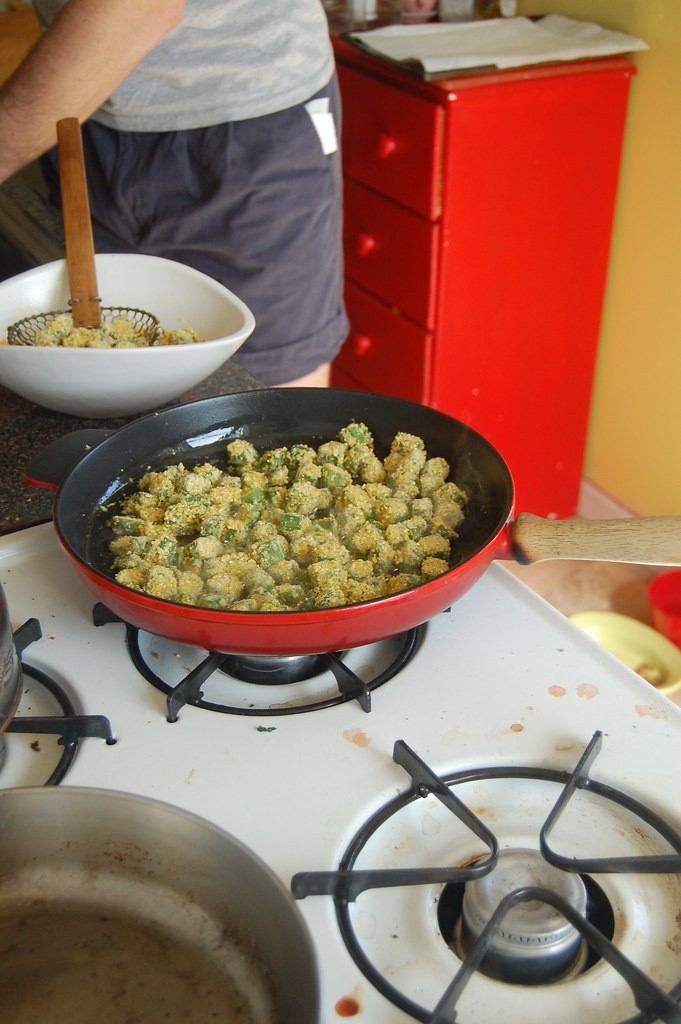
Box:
[330,25,639,555]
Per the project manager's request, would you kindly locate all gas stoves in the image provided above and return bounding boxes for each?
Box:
[0,561,681,1024]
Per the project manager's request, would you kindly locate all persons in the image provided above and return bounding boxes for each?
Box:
[1,0,349,390]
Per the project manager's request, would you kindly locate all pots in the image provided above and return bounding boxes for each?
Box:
[21,387,516,653]
[0,787,320,1024]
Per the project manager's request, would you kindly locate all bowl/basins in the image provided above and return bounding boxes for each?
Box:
[0,253,256,420]
[567,612,681,693]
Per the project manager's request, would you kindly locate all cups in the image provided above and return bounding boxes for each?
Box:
[648,569,681,652]
